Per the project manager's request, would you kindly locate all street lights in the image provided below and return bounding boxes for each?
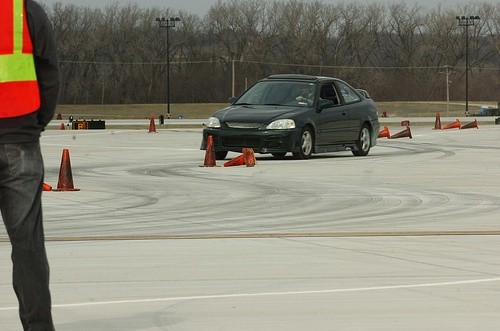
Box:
[154,15,181,120]
[455,15,481,115]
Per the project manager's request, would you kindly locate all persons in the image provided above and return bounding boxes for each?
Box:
[0,0,59,331]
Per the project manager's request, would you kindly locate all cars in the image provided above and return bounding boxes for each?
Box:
[203,74,379,160]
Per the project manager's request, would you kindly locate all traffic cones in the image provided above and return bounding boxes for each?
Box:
[198,135,220,168]
[378,126,391,139]
[41,184,52,191]
[432,112,443,130]
[59,123,65,130]
[147,115,158,133]
[390,126,413,139]
[50,149,81,191]
[225,148,257,168]
[400,121,410,127]
[443,119,461,130]
[462,119,479,129]
